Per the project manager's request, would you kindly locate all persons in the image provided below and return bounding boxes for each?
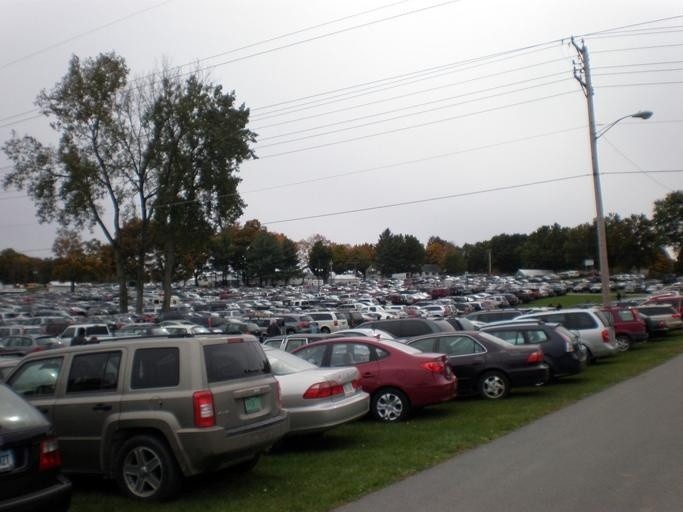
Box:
[68,325,97,344]
[549,301,562,311]
[614,290,627,300]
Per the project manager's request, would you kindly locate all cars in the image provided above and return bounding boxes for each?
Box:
[404,330,549,402]
[0,380,73,512]
[288,336,457,423]
[260,342,370,453]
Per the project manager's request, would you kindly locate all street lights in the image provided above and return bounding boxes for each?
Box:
[485,248,491,273]
[590,110,653,349]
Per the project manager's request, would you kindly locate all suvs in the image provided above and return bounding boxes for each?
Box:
[3,333,291,501]
